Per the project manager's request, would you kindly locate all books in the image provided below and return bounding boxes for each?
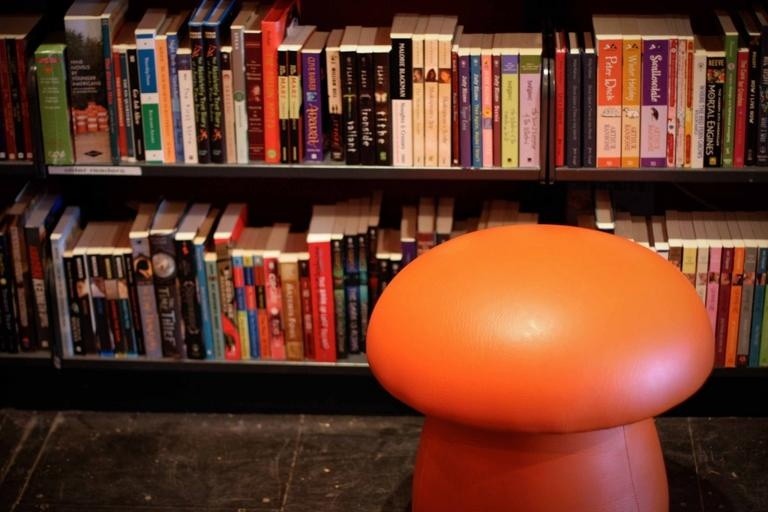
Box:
[434,185,669,259]
[665,206,768,369]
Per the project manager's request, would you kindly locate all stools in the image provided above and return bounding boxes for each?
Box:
[365,224,714,512]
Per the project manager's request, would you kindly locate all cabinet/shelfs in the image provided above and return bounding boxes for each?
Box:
[0,0,768,382]
